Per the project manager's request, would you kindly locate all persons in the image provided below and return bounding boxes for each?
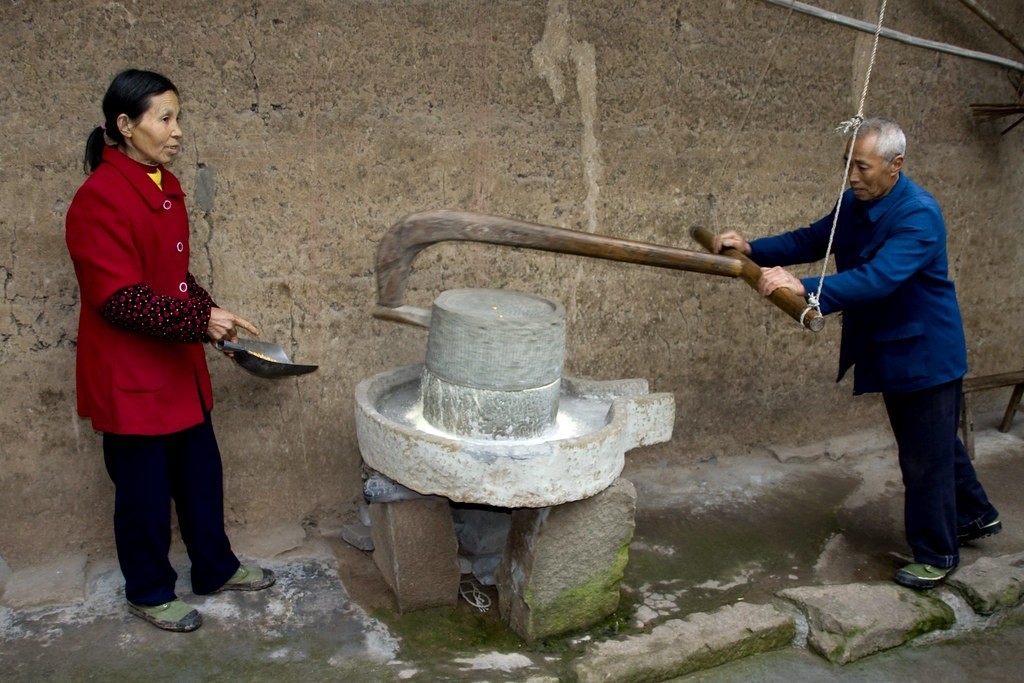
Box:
[64,67,278,632]
[709,117,1003,591]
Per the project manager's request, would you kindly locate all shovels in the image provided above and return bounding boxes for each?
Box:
[214,338,318,379]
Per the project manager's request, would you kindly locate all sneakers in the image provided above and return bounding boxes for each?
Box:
[126,596,204,633]
[954,515,1004,546]
[895,562,958,589]
[210,564,276,594]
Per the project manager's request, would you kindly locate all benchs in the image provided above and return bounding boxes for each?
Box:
[956,369,1023,460]
